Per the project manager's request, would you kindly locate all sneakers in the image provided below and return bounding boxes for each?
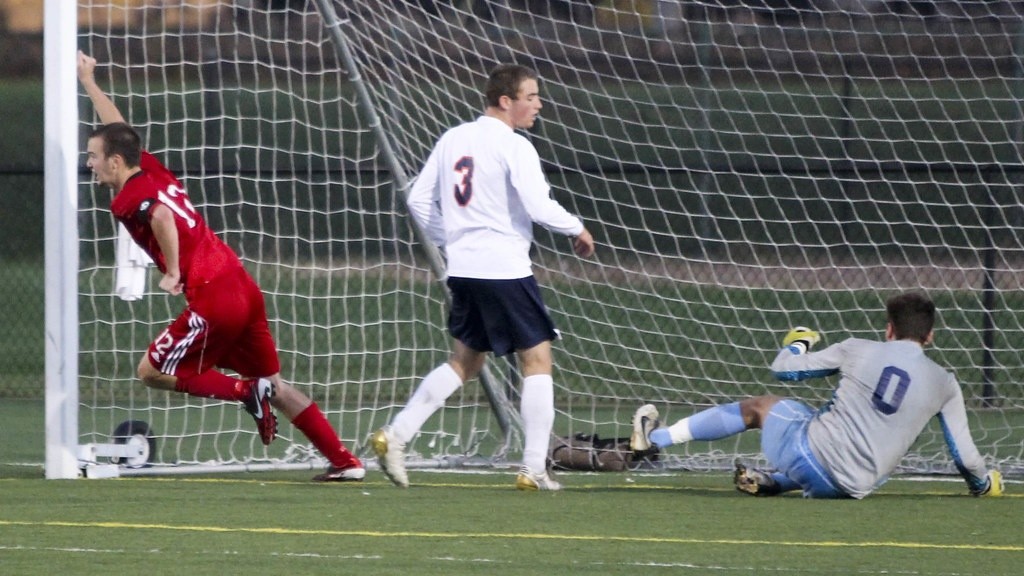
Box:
[733,462,780,497]
[517,465,560,491]
[629,404,659,457]
[244,377,278,445]
[312,457,365,482]
[372,425,409,488]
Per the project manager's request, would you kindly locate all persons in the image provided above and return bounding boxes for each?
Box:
[630,294,1004,498]
[373,64,595,491]
[77,50,366,481]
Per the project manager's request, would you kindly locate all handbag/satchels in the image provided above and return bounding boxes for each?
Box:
[550,430,659,471]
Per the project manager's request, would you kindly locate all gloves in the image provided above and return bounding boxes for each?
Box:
[783,327,821,351]
[969,470,1005,497]
